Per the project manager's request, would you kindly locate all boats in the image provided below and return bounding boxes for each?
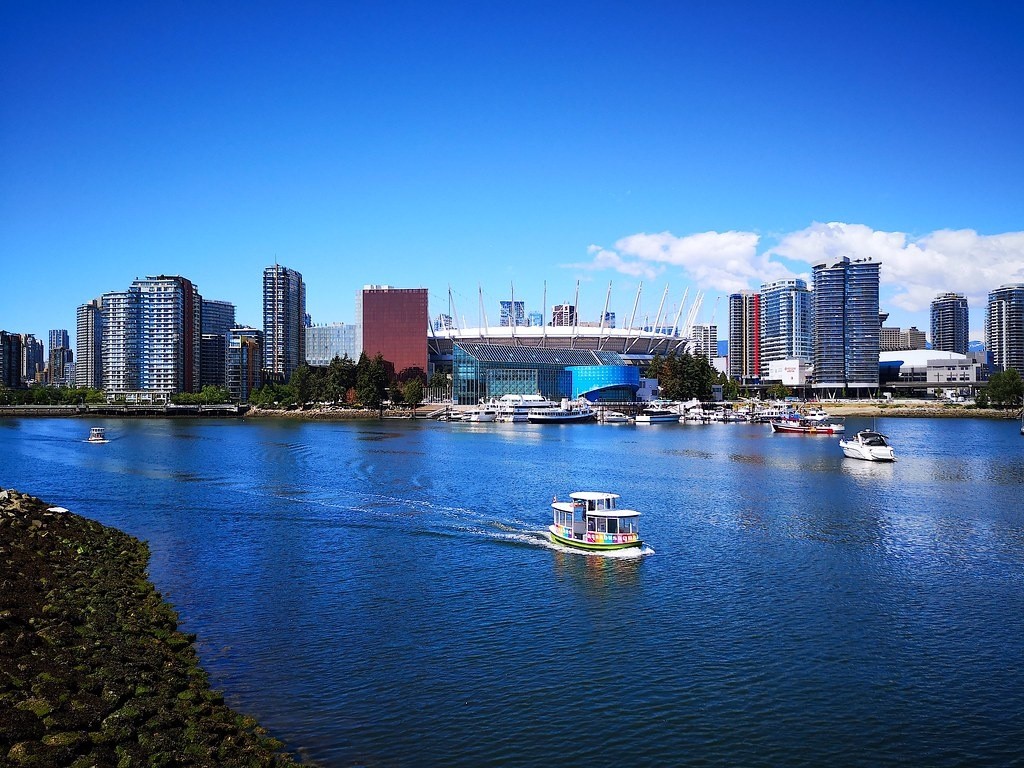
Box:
[436,398,501,422]
[606,403,829,423]
[769,411,845,435]
[495,393,558,422]
[546,489,655,560]
[82,428,111,443]
[839,429,899,463]
[528,397,598,424]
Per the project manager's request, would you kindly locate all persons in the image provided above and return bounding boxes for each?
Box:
[875,438,878,441]
[582,500,598,531]
[479,395,495,407]
[389,402,405,411]
